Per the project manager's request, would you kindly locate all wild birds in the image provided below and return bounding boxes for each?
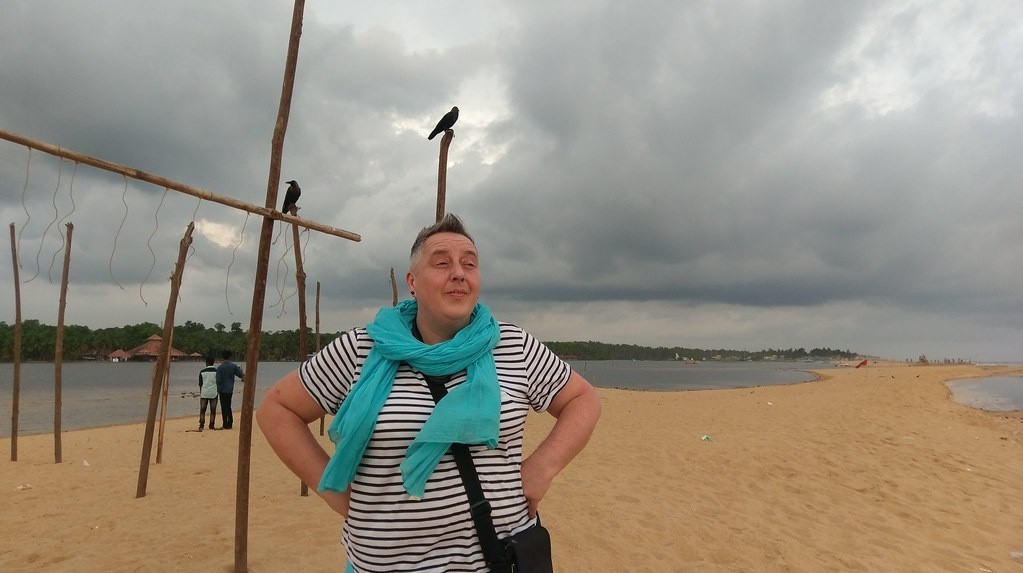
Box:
[282,180,302,214]
[428,106,459,140]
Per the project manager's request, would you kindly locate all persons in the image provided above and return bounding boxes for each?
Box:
[216,351,245,429]
[199,357,218,431]
[256,212,600,573]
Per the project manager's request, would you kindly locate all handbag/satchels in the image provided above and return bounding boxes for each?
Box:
[482,525,556,573]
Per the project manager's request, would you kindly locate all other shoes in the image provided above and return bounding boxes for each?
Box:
[209,426,216,431]
[220,426,232,430]
[198,427,203,432]
